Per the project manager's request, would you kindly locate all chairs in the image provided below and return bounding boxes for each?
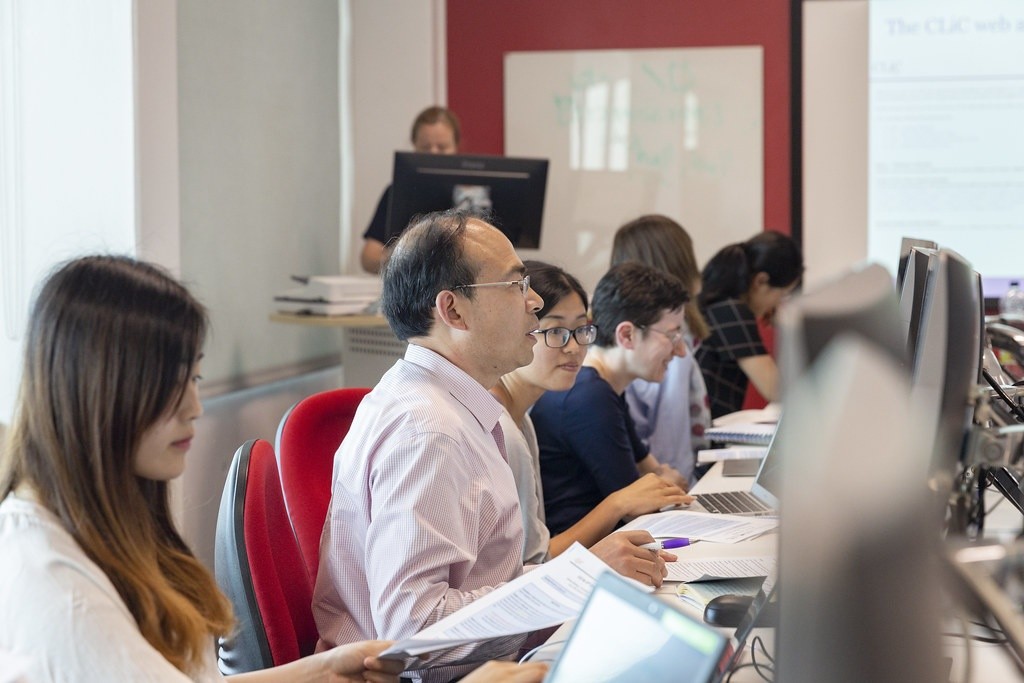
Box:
[210,387,373,675]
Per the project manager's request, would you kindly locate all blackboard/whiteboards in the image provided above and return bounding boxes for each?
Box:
[175,0,353,413]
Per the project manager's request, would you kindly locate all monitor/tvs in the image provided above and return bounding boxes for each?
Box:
[385,150,549,250]
[889,240,987,534]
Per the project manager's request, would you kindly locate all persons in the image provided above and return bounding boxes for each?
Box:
[610,214,713,502]
[696,230,806,420]
[530,263,686,538]
[311,208,677,683]
[361,107,461,271]
[488,259,698,563]
[0,258,549,683]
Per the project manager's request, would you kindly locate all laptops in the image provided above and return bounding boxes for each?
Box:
[663,419,781,517]
[539,571,734,683]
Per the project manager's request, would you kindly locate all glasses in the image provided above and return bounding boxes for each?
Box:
[613,322,684,347]
[531,324,599,348]
[434,275,531,298]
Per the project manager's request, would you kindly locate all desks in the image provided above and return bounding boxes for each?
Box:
[527,403,1024,683]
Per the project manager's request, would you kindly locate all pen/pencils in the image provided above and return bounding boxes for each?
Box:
[639,537,700,550]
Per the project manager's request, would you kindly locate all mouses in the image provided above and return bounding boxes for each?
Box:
[702,594,778,627]
